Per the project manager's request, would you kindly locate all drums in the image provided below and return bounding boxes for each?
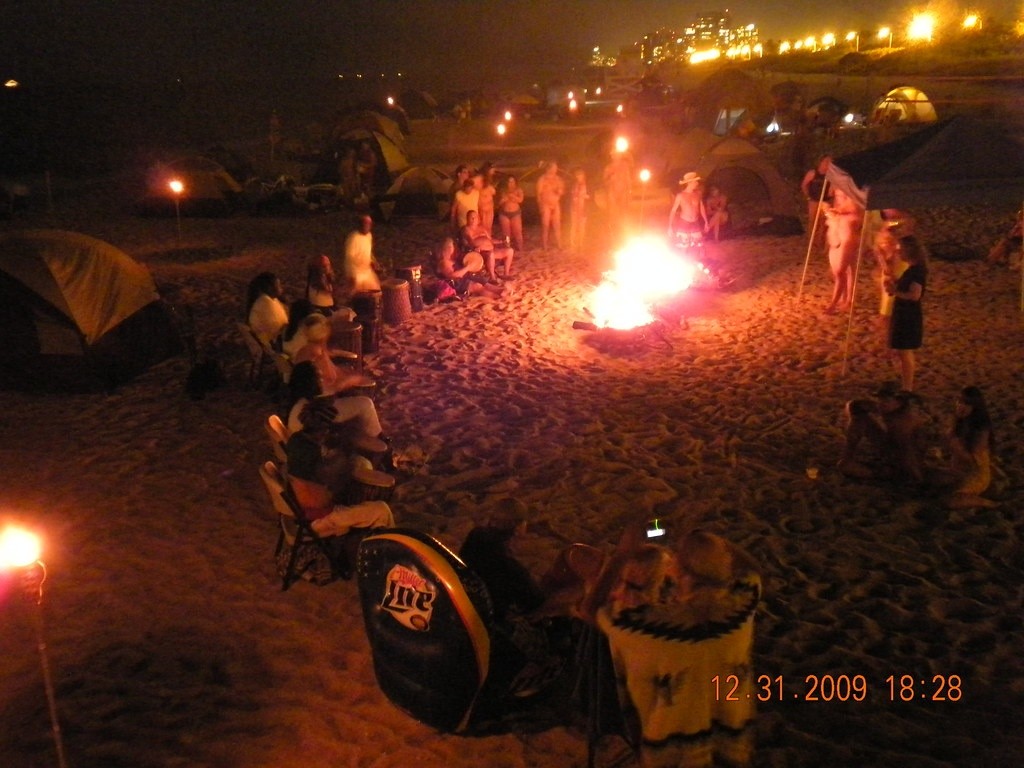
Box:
[345,462,397,516]
[463,251,485,275]
[345,432,389,472]
[397,263,425,316]
[348,287,383,354]
[340,375,378,402]
[380,279,413,325]
[324,318,365,377]
[472,235,495,253]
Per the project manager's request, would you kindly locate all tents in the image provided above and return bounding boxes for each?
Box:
[650,124,796,224]
[691,67,849,139]
[0,226,170,390]
[148,151,244,215]
[318,88,456,217]
[867,84,940,123]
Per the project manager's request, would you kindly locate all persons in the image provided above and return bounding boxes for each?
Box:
[803,155,927,392]
[836,381,1015,517]
[246,213,757,660]
[430,155,625,298]
[987,209,1024,265]
[667,172,730,261]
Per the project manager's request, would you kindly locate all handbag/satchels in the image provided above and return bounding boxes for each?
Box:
[277,545,331,586]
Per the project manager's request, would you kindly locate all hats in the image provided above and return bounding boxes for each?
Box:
[679,172,700,184]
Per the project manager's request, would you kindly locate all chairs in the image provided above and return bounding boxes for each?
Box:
[236,320,276,389]
[456,231,502,278]
[269,350,295,416]
[356,525,547,734]
[264,414,289,466]
[596,569,761,743]
[260,461,353,590]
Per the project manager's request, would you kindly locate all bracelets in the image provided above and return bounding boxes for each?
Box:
[806,198,811,200]
